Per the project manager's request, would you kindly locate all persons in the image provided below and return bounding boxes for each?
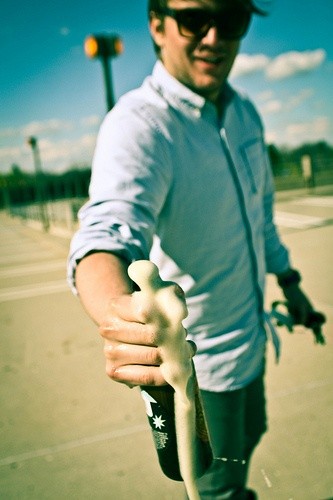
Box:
[66,0,328,500]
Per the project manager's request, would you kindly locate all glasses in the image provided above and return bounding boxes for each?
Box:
[160,4,254,40]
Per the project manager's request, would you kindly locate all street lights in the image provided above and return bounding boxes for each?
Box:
[27,135,51,230]
[85,32,124,113]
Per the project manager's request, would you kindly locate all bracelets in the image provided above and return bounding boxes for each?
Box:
[279,270,302,290]
